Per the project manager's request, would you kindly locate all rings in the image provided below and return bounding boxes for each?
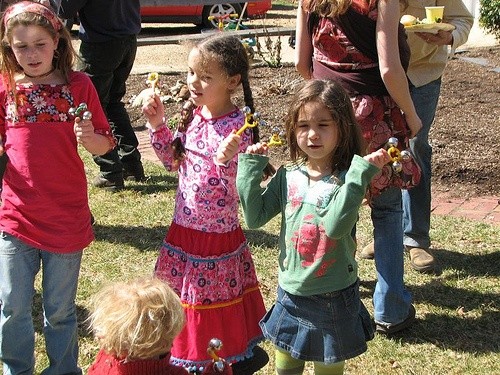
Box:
[425,39,429,42]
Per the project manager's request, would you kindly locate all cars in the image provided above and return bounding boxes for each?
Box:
[35,0,272,29]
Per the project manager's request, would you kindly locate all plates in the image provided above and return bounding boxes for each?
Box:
[403,23,456,34]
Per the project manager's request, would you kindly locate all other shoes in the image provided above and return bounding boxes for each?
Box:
[404,245,434,272]
[376,304,416,333]
[359,241,375,259]
[122,164,145,181]
[91,174,124,191]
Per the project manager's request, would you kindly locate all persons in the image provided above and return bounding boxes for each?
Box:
[294,0,422,334]
[85,276,233,375]
[360,0,475,270]
[0,0,146,375]
[235,78,391,375]
[142,33,276,375]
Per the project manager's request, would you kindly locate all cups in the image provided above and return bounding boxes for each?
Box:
[425,6,445,23]
